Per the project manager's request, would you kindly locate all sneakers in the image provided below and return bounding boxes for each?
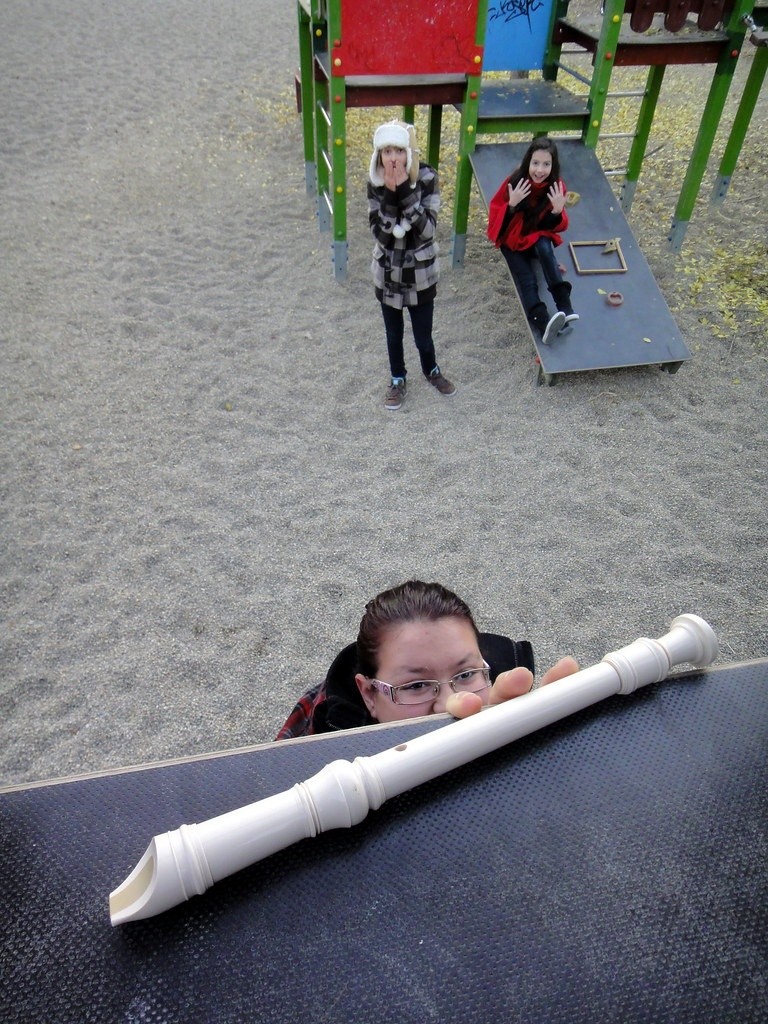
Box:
[385,378,408,410]
[422,364,456,396]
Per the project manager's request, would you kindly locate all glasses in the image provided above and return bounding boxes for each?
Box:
[371,658,491,705]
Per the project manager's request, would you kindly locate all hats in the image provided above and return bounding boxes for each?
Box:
[369,120,420,238]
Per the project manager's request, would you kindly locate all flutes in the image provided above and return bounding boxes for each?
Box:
[103,613,719,928]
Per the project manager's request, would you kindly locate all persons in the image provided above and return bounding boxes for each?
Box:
[275,580,582,744]
[487,137,579,346]
[366,119,457,410]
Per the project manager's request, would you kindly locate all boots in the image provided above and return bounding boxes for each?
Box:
[547,281,579,323]
[528,302,565,344]
